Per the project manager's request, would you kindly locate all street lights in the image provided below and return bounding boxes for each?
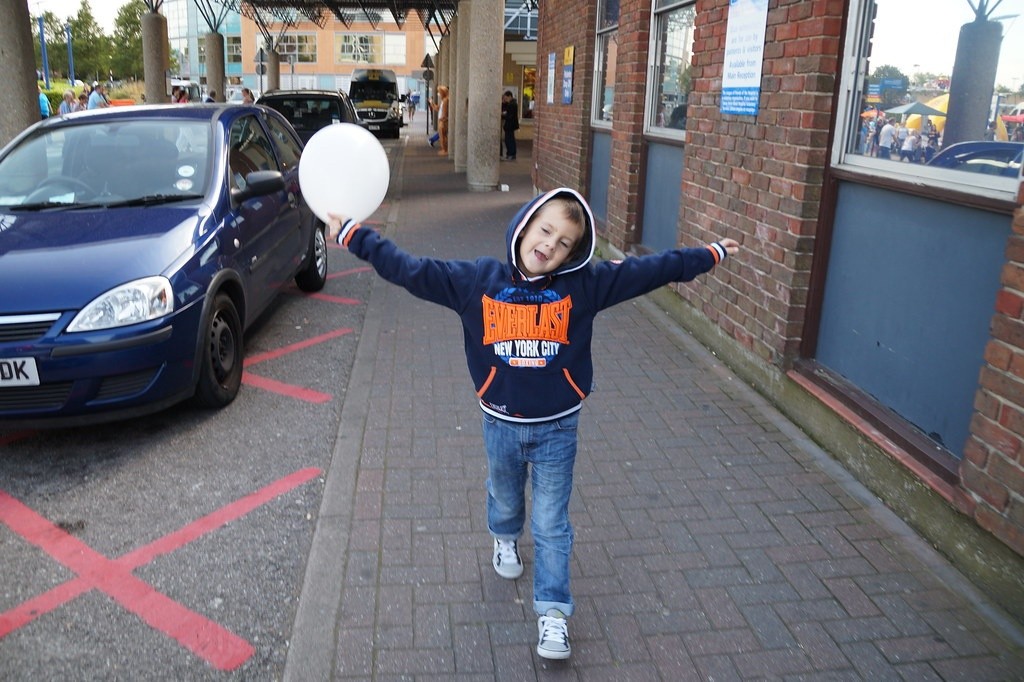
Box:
[36,12,50,92]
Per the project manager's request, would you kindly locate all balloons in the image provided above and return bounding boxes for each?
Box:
[298,122,390,225]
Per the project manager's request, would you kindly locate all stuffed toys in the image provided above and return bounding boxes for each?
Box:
[428,85,449,156]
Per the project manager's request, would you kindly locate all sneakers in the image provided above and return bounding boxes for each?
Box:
[535,614,573,660]
[492,538,525,579]
[428,138,434,148]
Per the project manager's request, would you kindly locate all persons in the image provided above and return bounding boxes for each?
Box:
[858,118,1024,162]
[171,86,255,104]
[37,81,112,120]
[500,91,521,161]
[428,132,439,148]
[327,188,740,659]
[406,88,415,122]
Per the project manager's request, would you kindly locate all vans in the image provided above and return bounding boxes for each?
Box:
[349,69,406,138]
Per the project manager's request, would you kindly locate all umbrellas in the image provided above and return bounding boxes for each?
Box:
[884,101,947,118]
[1001,114,1024,123]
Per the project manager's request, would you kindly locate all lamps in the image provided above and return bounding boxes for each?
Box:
[397,12,406,23]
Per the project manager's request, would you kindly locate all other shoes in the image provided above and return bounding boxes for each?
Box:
[506,155,517,161]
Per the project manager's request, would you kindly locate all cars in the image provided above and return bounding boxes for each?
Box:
[0,104,325,429]
[240,89,369,150]
